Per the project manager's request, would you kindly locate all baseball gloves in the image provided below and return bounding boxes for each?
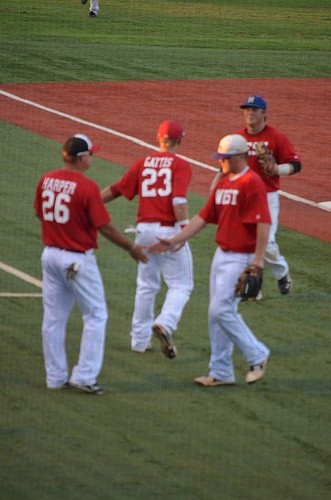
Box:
[235,265,263,302]
[255,141,276,177]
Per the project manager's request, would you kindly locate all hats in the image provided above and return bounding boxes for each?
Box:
[158,121,181,139]
[239,96,266,109]
[62,135,101,155]
[211,133,248,158]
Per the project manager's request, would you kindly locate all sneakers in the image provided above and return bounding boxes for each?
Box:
[277,271,291,295]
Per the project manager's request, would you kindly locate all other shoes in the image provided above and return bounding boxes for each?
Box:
[68,380,104,395]
[246,350,270,384]
[90,9,97,15]
[152,325,178,358]
[81,0,87,5]
[194,375,235,385]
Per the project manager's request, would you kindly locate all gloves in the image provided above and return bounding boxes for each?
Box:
[66,262,80,279]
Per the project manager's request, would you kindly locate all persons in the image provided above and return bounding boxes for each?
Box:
[96,120,191,361]
[209,95,301,301]
[81,0,99,16]
[147,134,272,387]
[33,133,150,395]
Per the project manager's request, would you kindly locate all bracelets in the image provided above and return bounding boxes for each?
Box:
[128,244,136,253]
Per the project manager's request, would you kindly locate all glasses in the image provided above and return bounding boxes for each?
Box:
[77,151,92,156]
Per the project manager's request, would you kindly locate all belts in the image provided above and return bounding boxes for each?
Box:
[62,248,86,253]
[160,222,185,228]
